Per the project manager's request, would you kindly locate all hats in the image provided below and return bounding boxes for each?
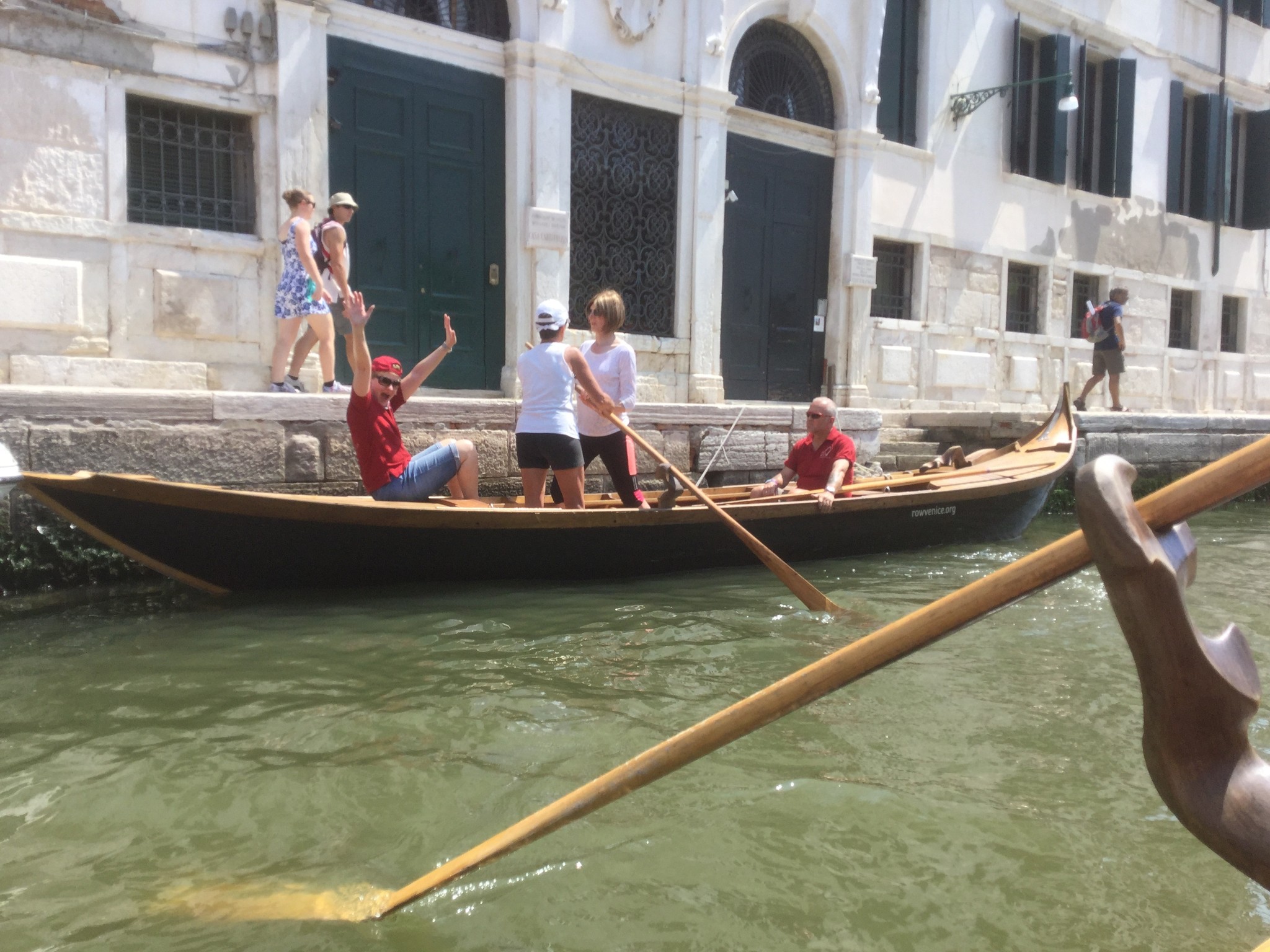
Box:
[328,193,358,208]
[372,355,402,378]
[535,298,571,324]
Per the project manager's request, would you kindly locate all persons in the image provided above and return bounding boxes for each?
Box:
[342,291,479,503]
[265,189,352,393]
[549,289,652,509]
[1072,287,1132,412]
[283,192,360,394]
[749,395,857,514]
[515,298,616,506]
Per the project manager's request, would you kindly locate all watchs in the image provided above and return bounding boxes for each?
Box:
[824,486,836,494]
[442,343,452,354]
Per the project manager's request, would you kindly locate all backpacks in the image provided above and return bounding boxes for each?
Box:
[1082,302,1114,343]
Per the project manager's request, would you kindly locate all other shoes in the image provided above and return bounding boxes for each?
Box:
[269,382,298,393]
[323,380,352,394]
[286,376,308,393]
[1074,398,1087,411]
[1113,404,1129,412]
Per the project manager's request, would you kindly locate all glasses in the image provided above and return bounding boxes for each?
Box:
[587,308,600,316]
[806,411,830,419]
[307,201,315,208]
[340,205,351,209]
[374,375,401,390]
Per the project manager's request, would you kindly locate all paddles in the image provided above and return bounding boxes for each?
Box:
[378,431,1269,926]
[521,339,842,613]
[716,459,1056,506]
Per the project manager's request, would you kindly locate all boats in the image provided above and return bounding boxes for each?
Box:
[15,380,1081,601]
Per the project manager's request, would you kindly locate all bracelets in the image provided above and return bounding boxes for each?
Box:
[765,479,778,486]
